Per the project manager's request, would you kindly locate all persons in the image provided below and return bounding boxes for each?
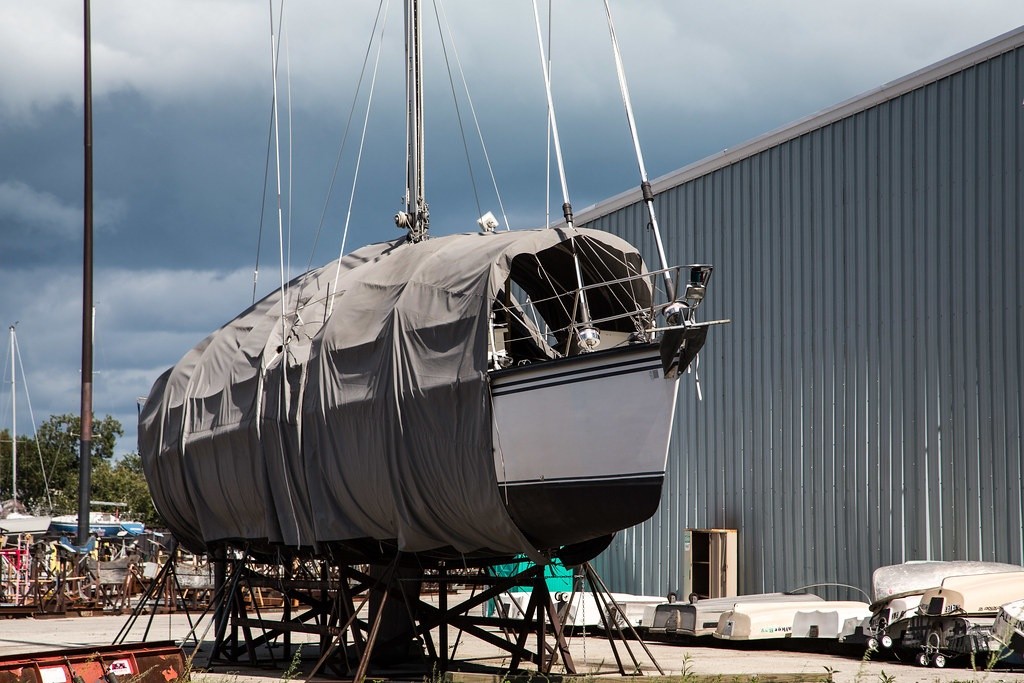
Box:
[689,593,698,605]
[666,592,677,604]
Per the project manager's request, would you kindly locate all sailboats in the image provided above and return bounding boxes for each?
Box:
[0,323,61,542]
[124,1,734,563]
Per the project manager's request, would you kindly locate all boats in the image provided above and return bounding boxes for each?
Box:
[47,514,147,541]
[492,572,1024,649]
